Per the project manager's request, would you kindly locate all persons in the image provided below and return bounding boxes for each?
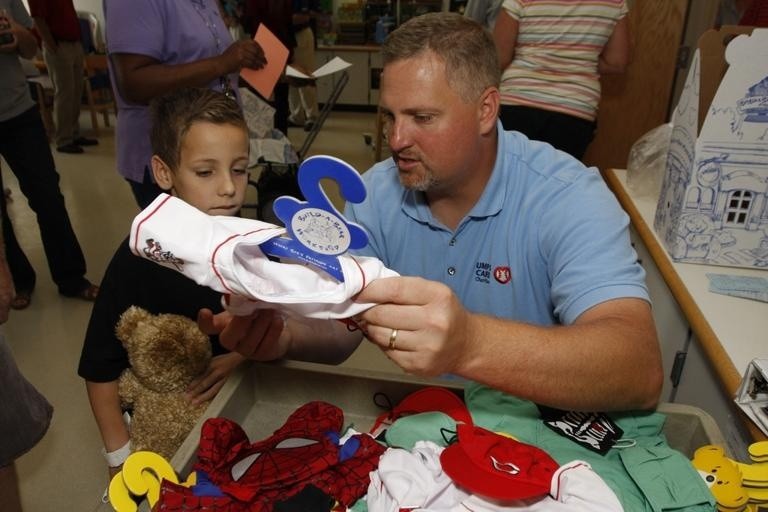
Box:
[78,87,252,510]
[197,12,665,414]
[26,0,101,154]
[285,0,321,131]
[0,233,54,512]
[2,0,99,310]
[490,0,632,163]
[235,0,297,136]
[105,1,270,211]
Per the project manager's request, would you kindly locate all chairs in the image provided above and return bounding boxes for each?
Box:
[35,83,57,139]
[82,77,118,129]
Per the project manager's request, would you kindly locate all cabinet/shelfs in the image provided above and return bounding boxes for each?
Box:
[627,224,753,467]
[314,51,387,106]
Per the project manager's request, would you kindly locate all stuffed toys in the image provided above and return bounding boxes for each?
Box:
[116,306,217,464]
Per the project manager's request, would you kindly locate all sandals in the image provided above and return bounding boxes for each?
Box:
[59,276,100,301]
[11,292,30,309]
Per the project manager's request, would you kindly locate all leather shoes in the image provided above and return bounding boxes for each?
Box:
[57,136,98,153]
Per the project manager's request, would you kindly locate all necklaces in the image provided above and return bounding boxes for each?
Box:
[192,0,231,93]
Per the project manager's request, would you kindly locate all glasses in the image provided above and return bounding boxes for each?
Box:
[220,76,238,101]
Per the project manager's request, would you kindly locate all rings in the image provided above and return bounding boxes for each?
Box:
[391,326,400,351]
